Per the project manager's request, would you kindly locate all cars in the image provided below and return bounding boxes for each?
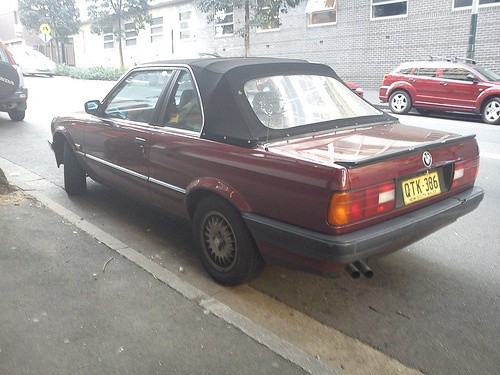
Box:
[51,57,484,288]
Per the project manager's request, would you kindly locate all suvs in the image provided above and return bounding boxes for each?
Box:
[0,40,27,121]
[379,54,500,125]
